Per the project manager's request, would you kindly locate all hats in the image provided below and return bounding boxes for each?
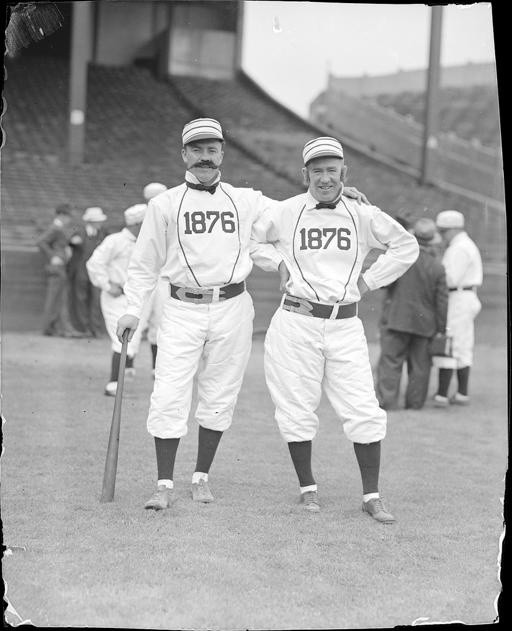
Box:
[142,181,168,202]
[181,117,225,148]
[435,209,466,230]
[303,136,345,165]
[56,202,75,218]
[81,207,108,224]
[405,217,443,249]
[122,205,148,228]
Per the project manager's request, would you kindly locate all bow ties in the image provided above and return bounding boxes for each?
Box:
[314,197,343,211]
[185,182,220,196]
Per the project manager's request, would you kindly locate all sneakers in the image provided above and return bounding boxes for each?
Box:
[424,395,448,410]
[144,485,175,512]
[298,491,320,513]
[104,366,137,397]
[189,476,214,505]
[449,394,471,405]
[361,492,395,527]
[42,326,103,339]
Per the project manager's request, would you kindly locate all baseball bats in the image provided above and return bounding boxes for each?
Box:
[101,329,129,503]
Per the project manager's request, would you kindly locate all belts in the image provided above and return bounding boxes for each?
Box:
[168,281,249,306]
[448,287,472,294]
[283,293,358,320]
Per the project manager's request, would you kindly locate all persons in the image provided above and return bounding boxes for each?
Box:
[69,207,110,337]
[250,137,419,524]
[142,182,167,378]
[372,209,483,409]
[115,118,372,510]
[38,203,84,338]
[86,203,148,396]
